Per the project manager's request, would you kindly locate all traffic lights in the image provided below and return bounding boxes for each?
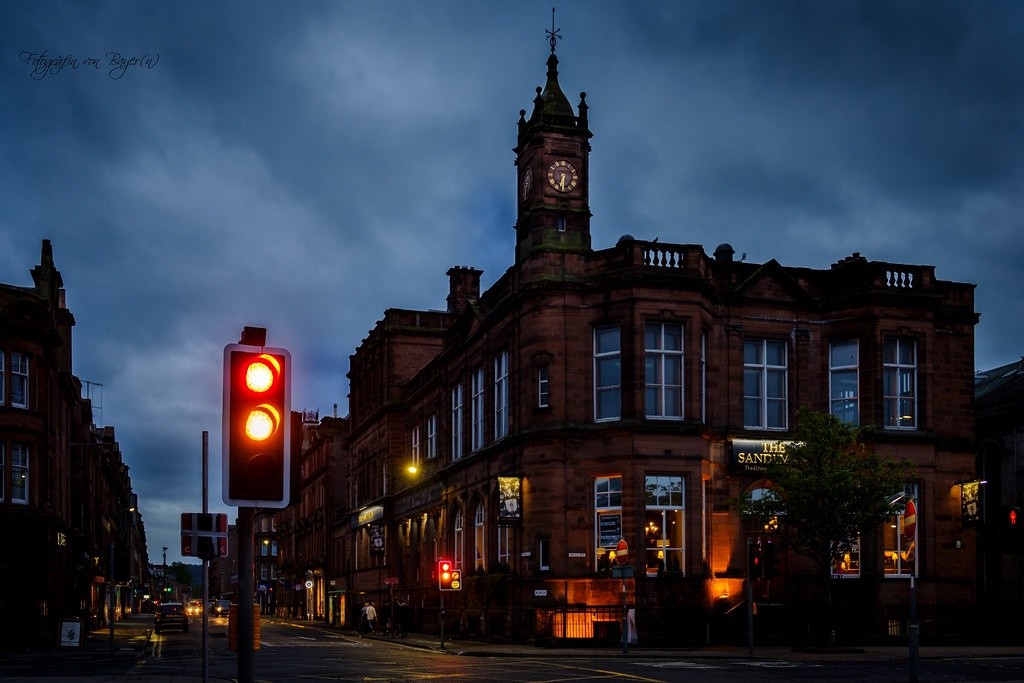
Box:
[222,342,293,509]
[439,560,463,592]
[1003,505,1022,530]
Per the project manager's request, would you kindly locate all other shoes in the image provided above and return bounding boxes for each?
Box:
[372,630,374,634]
[386,631,389,636]
[382,634,385,635]
[368,633,371,635]
[353,630,358,634]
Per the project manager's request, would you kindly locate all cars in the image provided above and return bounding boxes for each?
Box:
[211,599,233,616]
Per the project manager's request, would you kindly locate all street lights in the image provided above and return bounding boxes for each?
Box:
[161,547,169,603]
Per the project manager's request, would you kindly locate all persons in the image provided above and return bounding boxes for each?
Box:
[349,600,413,639]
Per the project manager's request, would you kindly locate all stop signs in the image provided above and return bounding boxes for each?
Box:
[616,538,629,566]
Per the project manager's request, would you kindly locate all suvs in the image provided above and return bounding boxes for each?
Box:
[154,601,190,633]
